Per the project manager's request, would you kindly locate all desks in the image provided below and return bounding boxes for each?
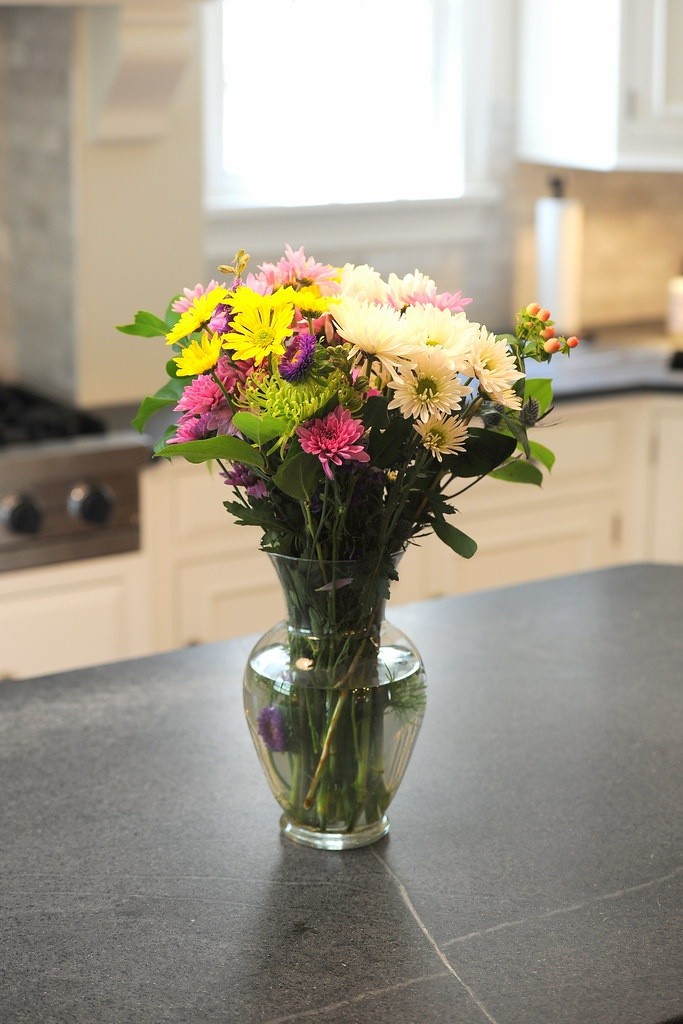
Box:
[0,561,683,1024]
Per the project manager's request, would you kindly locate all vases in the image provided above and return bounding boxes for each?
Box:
[243,539,427,851]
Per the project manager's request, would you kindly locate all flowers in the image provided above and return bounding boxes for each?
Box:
[115,241,578,560]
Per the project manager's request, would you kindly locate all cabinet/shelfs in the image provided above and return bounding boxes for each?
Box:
[644,393,683,563]
[514,0,683,176]
[0,551,164,681]
[136,393,653,652]
